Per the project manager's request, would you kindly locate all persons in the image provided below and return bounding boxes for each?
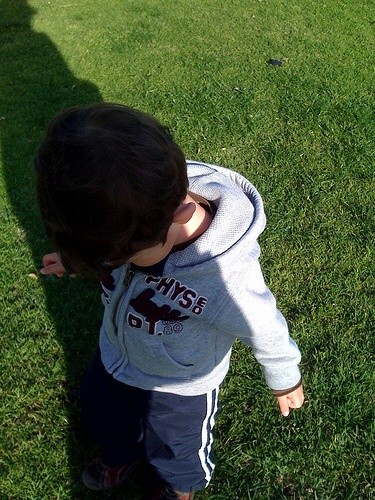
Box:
[37,99,305,500]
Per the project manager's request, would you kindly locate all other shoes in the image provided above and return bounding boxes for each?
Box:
[82,459,135,492]
[153,488,195,500]
